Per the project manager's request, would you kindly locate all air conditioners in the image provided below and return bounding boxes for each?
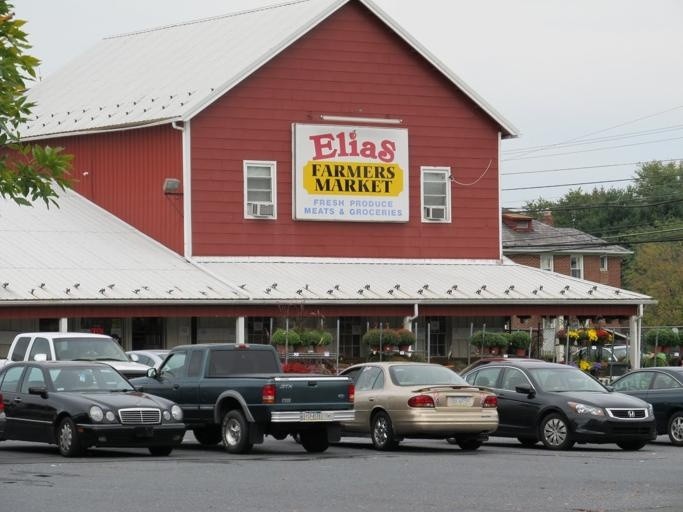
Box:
[424,206,446,220]
[250,202,274,219]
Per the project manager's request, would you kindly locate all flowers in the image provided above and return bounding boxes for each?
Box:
[555,327,613,373]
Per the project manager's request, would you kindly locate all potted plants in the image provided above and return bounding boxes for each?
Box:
[468,330,531,357]
[271,326,332,354]
[362,328,415,353]
[643,327,682,353]
